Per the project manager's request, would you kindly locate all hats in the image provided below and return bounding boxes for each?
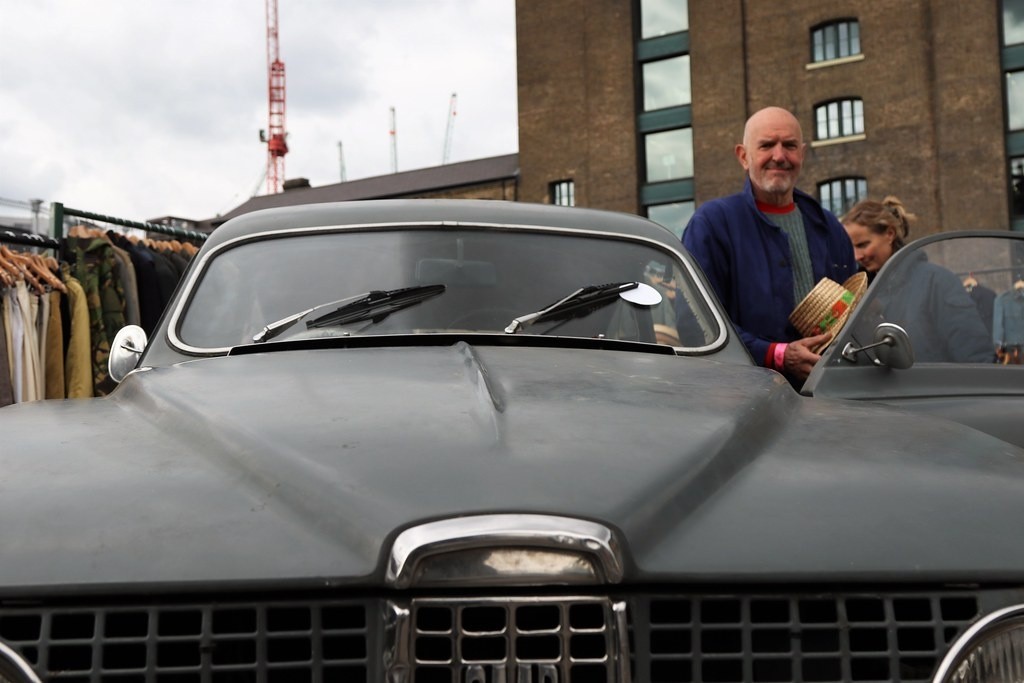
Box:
[788,271,868,354]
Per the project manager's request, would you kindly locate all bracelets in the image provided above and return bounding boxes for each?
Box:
[773,342,789,370]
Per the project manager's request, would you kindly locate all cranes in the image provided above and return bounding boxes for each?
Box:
[251,0,291,193]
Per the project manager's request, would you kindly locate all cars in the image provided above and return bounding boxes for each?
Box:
[0,194,1024,683]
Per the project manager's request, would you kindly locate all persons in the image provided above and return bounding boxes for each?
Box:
[961,276,1024,366]
[832,196,998,368]
[672,107,858,398]
